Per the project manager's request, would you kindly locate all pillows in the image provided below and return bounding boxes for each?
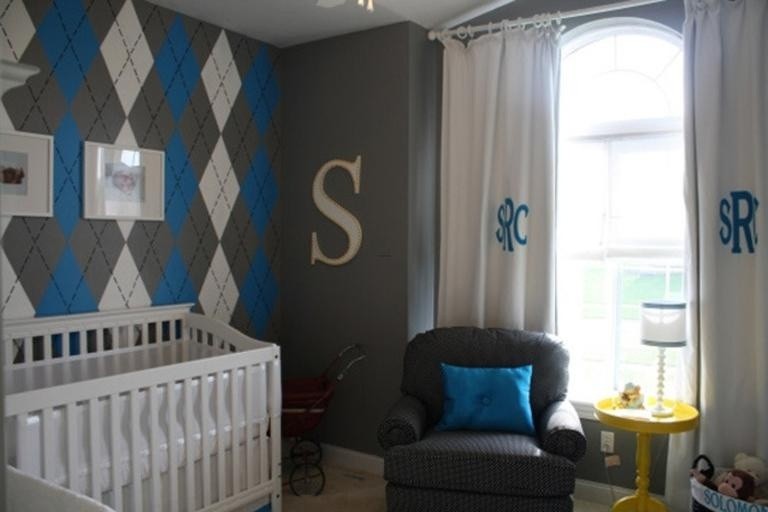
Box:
[433,361,536,435]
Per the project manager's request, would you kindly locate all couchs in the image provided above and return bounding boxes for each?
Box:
[377,325,588,512]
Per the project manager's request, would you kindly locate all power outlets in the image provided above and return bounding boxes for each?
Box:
[599,429,615,455]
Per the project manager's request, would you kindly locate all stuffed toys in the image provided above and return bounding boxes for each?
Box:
[688,454,755,502]
[714,453,767,503]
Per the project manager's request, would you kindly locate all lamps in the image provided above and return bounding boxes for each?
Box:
[641,300,689,417]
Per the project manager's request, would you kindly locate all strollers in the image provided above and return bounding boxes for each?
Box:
[282,343,369,496]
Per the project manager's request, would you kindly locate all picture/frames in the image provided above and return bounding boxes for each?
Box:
[0,129,54,218]
[82,140,166,222]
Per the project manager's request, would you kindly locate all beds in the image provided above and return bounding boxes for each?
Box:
[2,303,283,512]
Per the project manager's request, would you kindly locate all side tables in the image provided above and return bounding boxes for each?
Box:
[593,396,700,512]
